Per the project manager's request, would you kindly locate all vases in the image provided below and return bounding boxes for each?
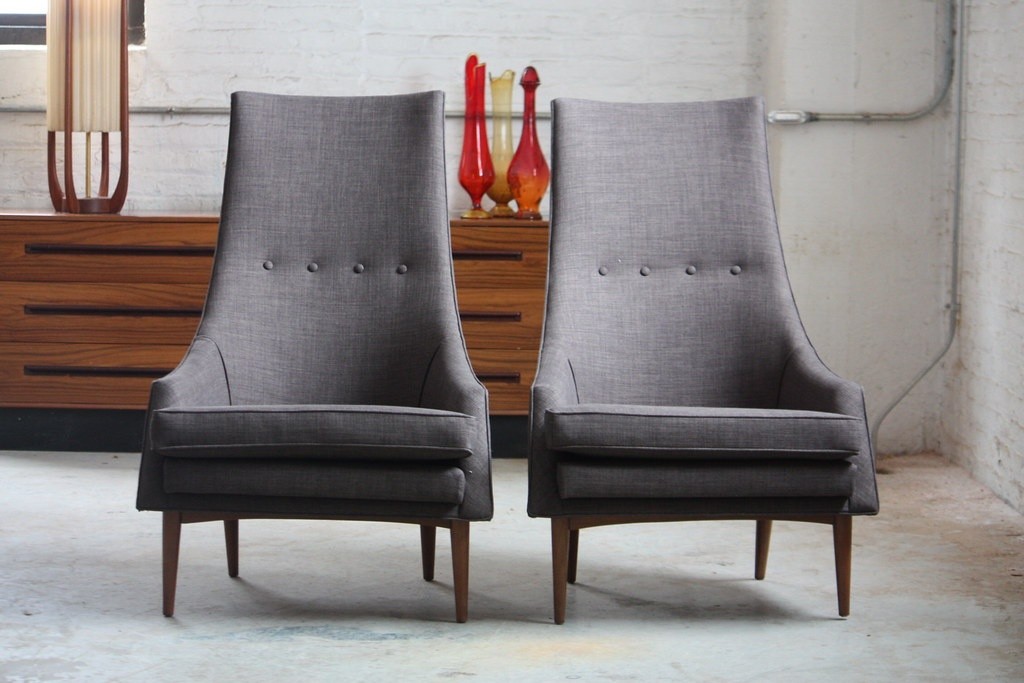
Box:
[458,53,552,221]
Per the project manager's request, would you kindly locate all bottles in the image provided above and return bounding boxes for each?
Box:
[485,67,515,218]
[506,66,550,219]
[457,51,496,220]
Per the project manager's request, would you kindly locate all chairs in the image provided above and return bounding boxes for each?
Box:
[135,90,495,623]
[528,95,880,624]
